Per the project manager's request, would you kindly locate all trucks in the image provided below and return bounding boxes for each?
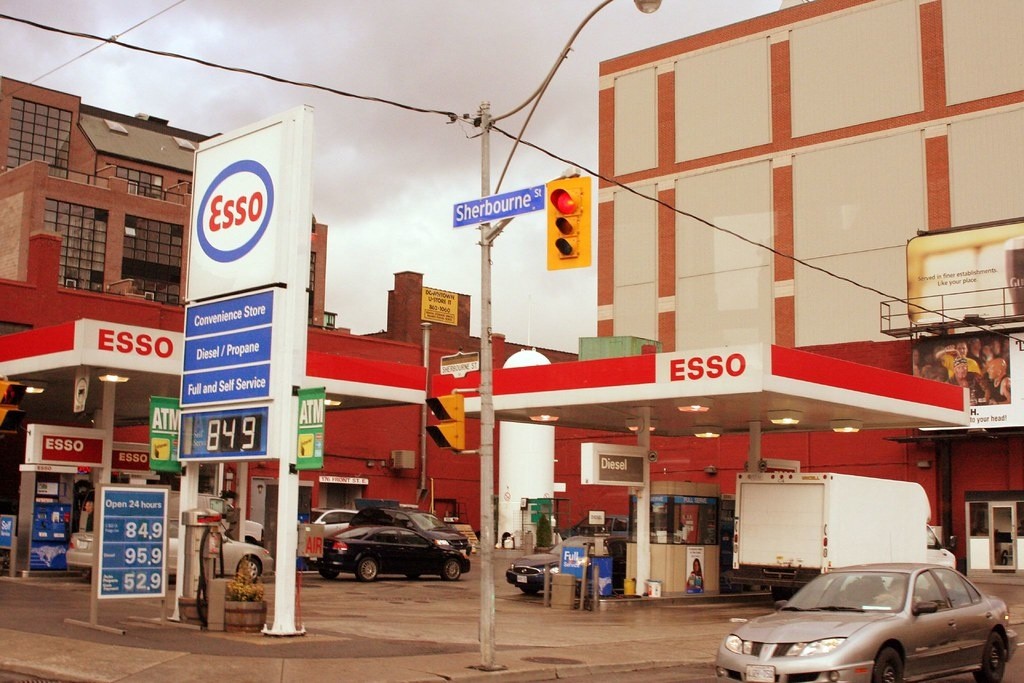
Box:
[731,473,956,604]
[79,491,265,548]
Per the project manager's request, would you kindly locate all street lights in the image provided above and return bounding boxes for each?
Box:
[481,0,662,671]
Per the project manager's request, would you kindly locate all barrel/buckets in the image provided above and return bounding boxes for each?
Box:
[624,578,636,595]
[647,581,661,597]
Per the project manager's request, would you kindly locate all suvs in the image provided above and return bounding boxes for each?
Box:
[348,506,472,555]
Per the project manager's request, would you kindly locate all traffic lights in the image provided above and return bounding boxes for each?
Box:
[545,177,592,271]
[0,381,28,436]
[424,394,466,452]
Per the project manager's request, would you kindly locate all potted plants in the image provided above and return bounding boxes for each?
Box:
[220,490,238,504]
[534,513,555,554]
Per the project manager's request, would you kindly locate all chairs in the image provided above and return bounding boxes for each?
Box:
[842,575,886,608]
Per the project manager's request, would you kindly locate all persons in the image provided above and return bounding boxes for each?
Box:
[686,558,704,589]
[911,336,1012,406]
[81,501,94,582]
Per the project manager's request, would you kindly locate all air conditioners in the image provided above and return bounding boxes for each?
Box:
[391,450,415,468]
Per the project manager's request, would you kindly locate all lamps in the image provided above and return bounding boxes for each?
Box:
[917,460,933,467]
[964,313,986,324]
[673,397,713,412]
[97,368,133,382]
[525,407,561,421]
[368,460,375,467]
[25,381,48,393]
[324,393,343,406]
[625,418,660,431]
[830,419,863,432]
[704,466,717,473]
[688,425,723,437]
[766,410,803,424]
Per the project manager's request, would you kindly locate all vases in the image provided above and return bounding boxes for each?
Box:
[179,597,209,625]
[224,602,267,633]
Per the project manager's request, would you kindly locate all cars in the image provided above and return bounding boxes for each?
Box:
[65,517,275,584]
[715,562,1019,683]
[311,507,359,538]
[305,524,471,583]
[505,534,628,595]
[560,514,630,541]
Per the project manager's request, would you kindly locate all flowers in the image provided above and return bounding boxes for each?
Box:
[225,553,266,602]
